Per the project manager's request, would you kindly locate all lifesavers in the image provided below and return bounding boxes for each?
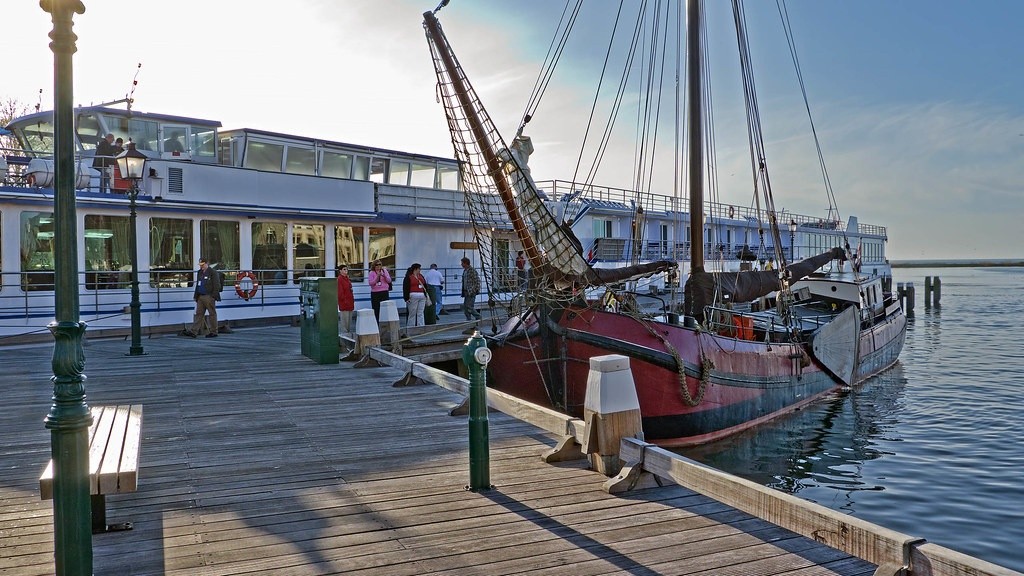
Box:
[235,272,258,300]
[719,317,743,340]
[729,206,734,217]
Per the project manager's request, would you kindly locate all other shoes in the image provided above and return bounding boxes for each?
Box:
[476,314,482,326]
[183,331,196,338]
[462,328,476,335]
[205,333,218,338]
[436,315,439,320]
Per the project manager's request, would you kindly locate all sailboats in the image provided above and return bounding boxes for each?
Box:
[421,1,906,446]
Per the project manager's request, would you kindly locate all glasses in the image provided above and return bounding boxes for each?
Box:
[375,264,381,266]
[344,269,348,270]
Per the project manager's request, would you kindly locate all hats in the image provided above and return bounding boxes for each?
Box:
[197,258,207,265]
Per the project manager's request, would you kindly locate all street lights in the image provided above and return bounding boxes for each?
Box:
[116,136,148,356]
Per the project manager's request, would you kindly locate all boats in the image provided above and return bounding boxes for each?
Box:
[0,61,894,353]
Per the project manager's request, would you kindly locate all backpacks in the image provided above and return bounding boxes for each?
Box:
[209,268,225,291]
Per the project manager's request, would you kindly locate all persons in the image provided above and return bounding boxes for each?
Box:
[92,134,125,193]
[338,265,354,333]
[516,251,526,294]
[185,258,222,338]
[164,131,184,152]
[368,260,392,326]
[460,258,481,334]
[765,257,774,271]
[424,264,444,320]
[274,266,287,285]
[403,263,427,326]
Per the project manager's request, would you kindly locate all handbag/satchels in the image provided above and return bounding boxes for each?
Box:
[425,293,432,306]
[389,283,392,291]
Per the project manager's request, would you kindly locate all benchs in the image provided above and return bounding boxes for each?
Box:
[38,403,144,532]
